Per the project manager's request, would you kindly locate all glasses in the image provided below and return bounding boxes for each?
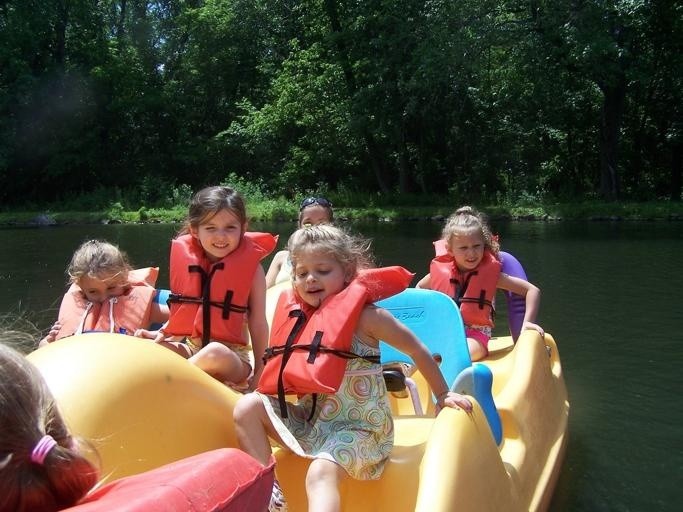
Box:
[299,196,330,212]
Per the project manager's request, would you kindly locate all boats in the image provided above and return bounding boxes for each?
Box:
[23,311,574,512]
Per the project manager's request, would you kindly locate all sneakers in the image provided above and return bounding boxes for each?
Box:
[268,479,293,511]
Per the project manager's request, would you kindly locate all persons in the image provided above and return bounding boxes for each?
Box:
[263,197,333,289]
[153,186,268,391]
[0,341,99,512]
[39,240,171,345]
[384,207,545,398]
[232,224,474,512]
